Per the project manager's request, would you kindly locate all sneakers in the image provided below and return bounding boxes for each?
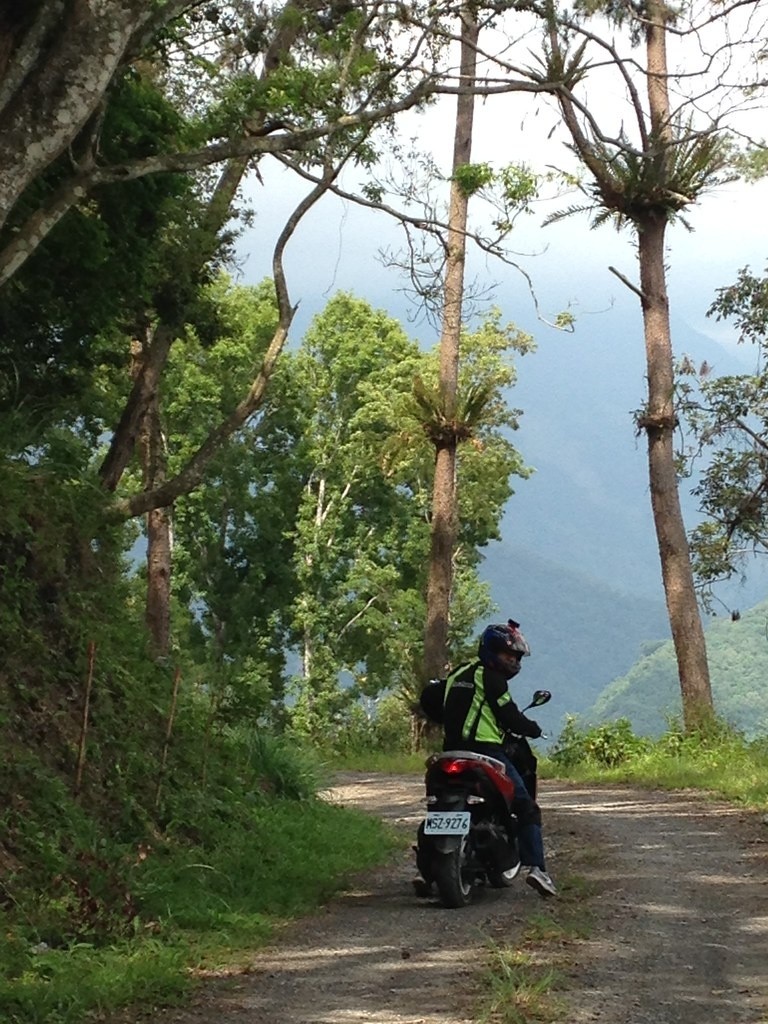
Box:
[412,872,429,898]
[526,866,557,896]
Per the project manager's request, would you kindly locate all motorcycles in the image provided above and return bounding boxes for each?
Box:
[411,690,552,909]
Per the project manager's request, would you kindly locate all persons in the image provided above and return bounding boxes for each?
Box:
[407,625,559,898]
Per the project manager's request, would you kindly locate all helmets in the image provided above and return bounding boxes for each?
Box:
[478,618,526,679]
